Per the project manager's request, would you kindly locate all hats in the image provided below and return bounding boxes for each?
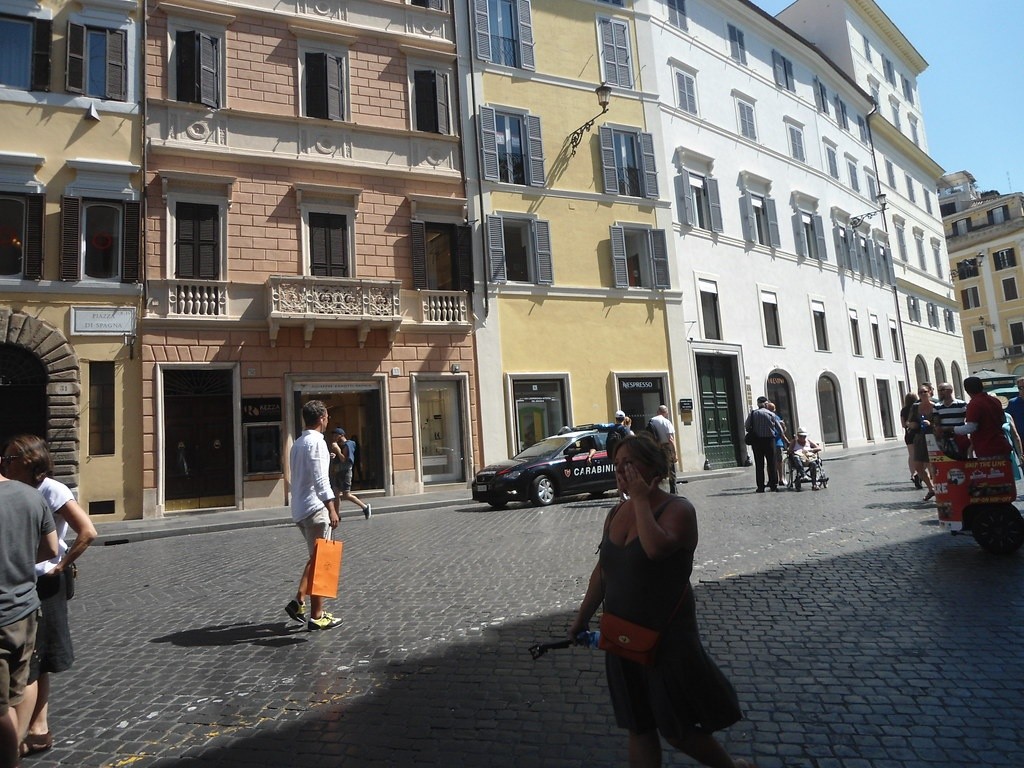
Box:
[332,428,346,436]
[757,396,768,404]
[986,392,1008,409]
[615,410,625,418]
[797,427,809,436]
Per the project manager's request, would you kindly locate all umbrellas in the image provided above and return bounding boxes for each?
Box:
[970,368,1020,385]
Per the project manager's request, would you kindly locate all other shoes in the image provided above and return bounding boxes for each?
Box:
[794,483,801,491]
[756,488,765,493]
[911,474,923,489]
[923,490,935,501]
[363,504,371,520]
[812,484,820,490]
[771,486,779,492]
[779,481,784,485]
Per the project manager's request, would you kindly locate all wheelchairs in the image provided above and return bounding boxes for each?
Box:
[782,444,829,491]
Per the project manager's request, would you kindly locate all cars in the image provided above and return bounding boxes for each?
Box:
[470,425,635,506]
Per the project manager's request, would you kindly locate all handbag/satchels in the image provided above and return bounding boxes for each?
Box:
[299,525,343,599]
[63,562,77,600]
[645,417,658,442]
[905,402,918,441]
[597,612,661,665]
[745,410,755,445]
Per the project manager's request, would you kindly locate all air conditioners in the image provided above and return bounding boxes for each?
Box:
[1005,345,1024,355]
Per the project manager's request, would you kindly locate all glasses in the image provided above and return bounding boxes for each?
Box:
[0,456,20,465]
[1018,386,1024,390]
[938,389,952,393]
[919,391,929,394]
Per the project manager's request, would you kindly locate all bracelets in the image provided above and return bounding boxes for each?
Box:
[588,456,591,458]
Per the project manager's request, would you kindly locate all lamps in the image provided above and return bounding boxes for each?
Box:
[568,81,613,156]
[950,252,984,281]
[851,190,887,228]
[979,315,996,332]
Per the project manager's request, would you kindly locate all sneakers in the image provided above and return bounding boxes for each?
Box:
[286,599,308,623]
[308,611,343,632]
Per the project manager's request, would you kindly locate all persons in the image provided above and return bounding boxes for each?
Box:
[0,471,58,768]
[564,432,747,768]
[329,427,371,520]
[581,406,677,503]
[900,378,1024,501]
[285,400,343,632]
[351,436,362,482]
[745,397,821,490]
[2,437,97,758]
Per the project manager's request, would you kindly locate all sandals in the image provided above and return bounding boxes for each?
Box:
[19,730,53,758]
